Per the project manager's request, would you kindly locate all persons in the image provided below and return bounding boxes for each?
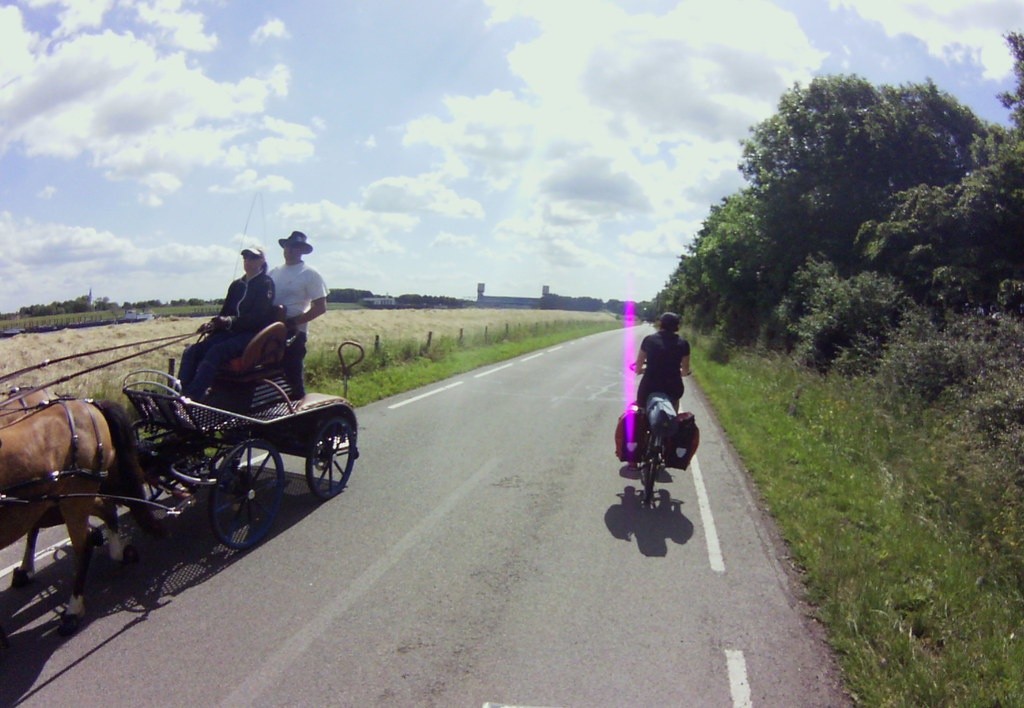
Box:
[628,313,691,473]
[173,247,275,410]
[267,232,330,402]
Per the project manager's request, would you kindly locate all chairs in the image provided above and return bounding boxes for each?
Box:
[196,305,288,375]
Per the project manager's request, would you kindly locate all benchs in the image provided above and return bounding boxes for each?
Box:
[242,354,293,414]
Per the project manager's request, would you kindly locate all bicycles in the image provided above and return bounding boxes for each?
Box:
[627,360,693,502]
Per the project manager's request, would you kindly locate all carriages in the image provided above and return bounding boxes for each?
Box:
[0,303,364,639]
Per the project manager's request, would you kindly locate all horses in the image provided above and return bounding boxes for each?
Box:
[0,387,154,636]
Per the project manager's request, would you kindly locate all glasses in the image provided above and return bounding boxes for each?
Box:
[242,252,260,260]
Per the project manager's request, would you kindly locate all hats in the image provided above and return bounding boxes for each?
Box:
[241,246,264,255]
[279,231,312,254]
[659,312,679,325]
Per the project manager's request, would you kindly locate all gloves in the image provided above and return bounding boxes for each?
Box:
[213,316,231,331]
[196,323,212,335]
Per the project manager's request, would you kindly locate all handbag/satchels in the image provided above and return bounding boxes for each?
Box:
[613,402,650,462]
[665,412,699,471]
